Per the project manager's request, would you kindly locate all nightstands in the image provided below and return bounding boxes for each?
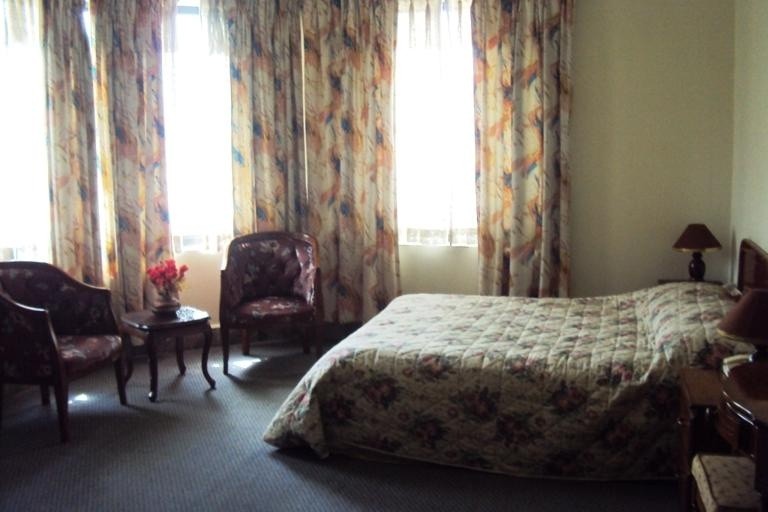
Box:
[706,371,768,512]
[657,278,723,285]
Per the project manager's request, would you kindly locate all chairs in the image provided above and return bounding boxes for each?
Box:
[0,261,128,444]
[219,230,324,375]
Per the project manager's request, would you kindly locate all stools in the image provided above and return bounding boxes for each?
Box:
[688,454,766,512]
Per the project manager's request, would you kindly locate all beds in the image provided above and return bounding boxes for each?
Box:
[261,237,768,487]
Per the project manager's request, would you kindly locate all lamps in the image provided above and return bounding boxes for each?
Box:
[672,224,722,281]
[715,288,767,384]
